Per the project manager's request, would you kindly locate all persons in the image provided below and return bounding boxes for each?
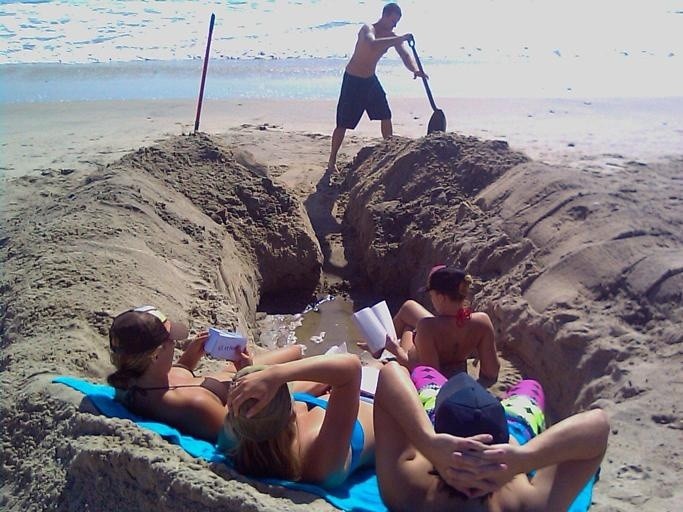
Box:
[106,302,332,441]
[212,347,402,488]
[366,359,612,512]
[355,263,502,390]
[322,1,430,178]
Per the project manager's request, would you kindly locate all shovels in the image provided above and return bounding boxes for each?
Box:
[407,34,446,135]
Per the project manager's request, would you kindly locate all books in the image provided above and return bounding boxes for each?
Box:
[359,366,380,398]
[350,298,399,353]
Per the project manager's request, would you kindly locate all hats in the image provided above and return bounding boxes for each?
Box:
[108,306,188,356]
[416,264,468,299]
[436,371,510,445]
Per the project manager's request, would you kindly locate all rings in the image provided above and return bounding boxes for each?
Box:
[232,381,241,387]
